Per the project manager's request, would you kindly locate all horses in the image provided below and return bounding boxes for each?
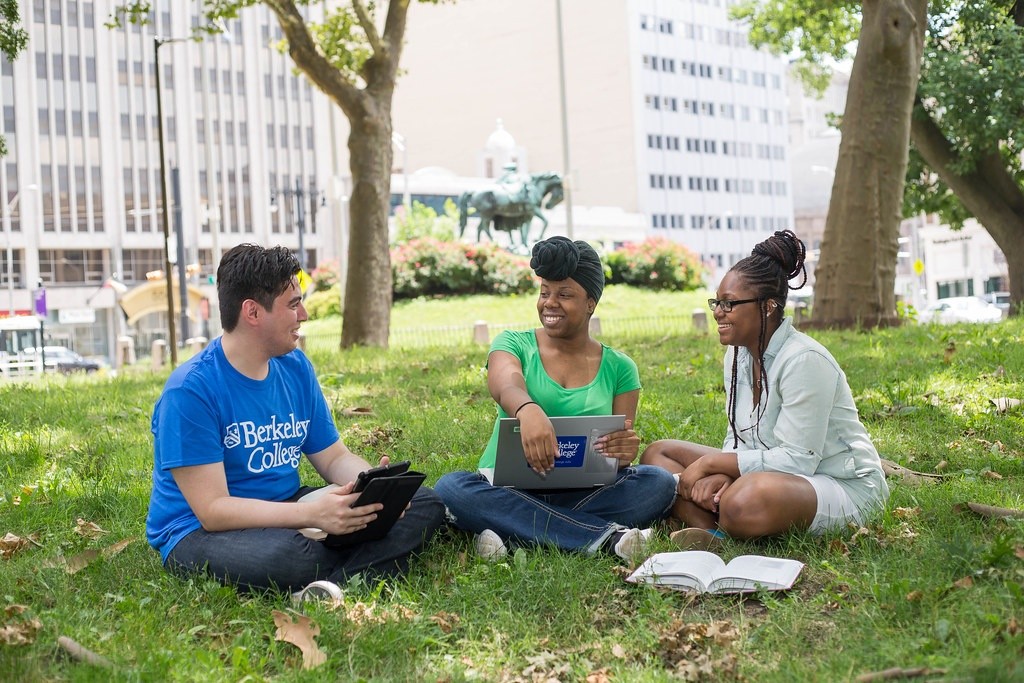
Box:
[459,172,564,255]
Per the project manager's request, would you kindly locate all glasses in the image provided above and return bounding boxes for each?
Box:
[707,297,766,313]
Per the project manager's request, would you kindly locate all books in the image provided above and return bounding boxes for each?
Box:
[624,550,804,597]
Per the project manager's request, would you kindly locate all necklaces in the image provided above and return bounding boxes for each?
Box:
[757,378,761,389]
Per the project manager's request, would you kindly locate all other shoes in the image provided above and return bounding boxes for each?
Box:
[290,580,344,613]
[608,525,655,567]
[471,528,507,562]
[670,526,732,551]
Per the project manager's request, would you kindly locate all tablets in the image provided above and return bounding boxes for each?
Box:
[350,460,412,494]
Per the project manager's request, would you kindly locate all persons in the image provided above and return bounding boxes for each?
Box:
[145,243,442,606]
[638,229,889,550]
[433,236,676,565]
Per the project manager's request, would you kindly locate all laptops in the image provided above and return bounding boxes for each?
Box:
[478,414,626,488]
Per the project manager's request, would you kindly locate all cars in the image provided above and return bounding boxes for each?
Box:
[915,295,1003,323]
[7,346,100,374]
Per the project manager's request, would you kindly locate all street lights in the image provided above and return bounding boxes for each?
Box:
[2,184,37,352]
[268,191,328,270]
[704,209,733,263]
[154,35,185,369]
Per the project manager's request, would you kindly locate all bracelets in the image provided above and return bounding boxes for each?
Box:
[515,401,537,419]
